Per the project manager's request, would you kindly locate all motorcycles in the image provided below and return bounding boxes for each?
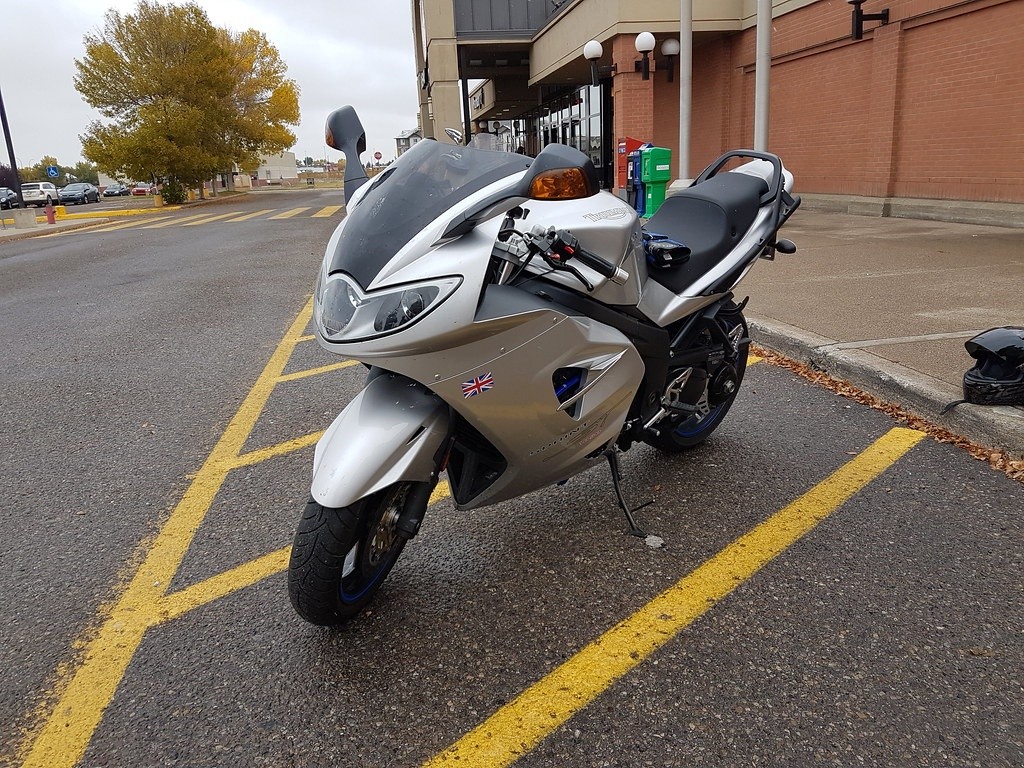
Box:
[288,106,801,628]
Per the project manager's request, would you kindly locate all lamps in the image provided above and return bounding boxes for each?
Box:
[478,121,487,133]
[513,121,528,137]
[635,32,656,80]
[584,40,617,87]
[654,37,680,82]
[488,122,500,136]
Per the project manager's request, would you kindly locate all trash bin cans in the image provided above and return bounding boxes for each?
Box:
[188,191,195,200]
[203,187,209,196]
[154,194,163,207]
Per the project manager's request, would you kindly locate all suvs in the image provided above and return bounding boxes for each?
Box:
[21,182,62,207]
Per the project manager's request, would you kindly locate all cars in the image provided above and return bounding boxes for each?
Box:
[103,183,131,197]
[0,187,20,209]
[58,183,101,206]
[132,182,150,195]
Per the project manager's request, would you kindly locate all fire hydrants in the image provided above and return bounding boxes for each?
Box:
[43,204,56,224]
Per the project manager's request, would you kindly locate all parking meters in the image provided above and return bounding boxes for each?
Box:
[626,142,672,219]
